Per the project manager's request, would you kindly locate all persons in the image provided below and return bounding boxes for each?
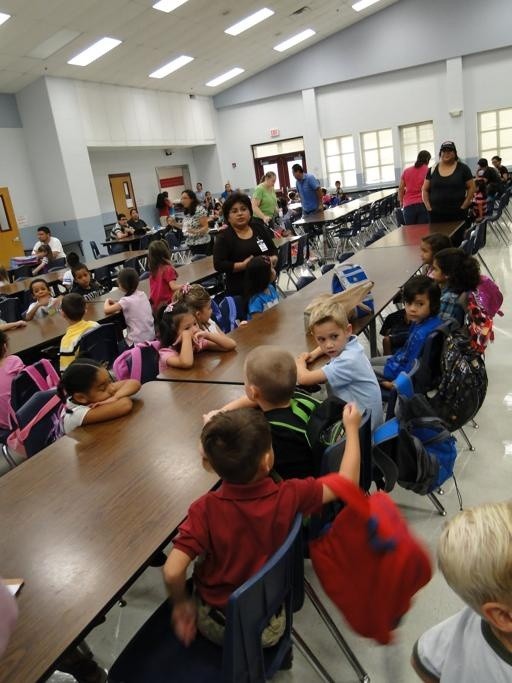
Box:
[54,356,146,435]
[400,142,512,332]
[1,225,103,332]
[103,267,158,350]
[199,345,351,538]
[156,304,243,371]
[292,301,387,466]
[367,274,444,412]
[408,498,511,682]
[56,291,107,376]
[0,329,30,450]
[112,182,279,322]
[160,400,362,672]
[252,163,344,265]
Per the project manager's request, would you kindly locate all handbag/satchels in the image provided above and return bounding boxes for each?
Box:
[7,428,27,458]
[331,263,375,318]
[469,277,503,314]
[463,305,493,354]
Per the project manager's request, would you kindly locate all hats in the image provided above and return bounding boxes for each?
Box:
[442,141,455,150]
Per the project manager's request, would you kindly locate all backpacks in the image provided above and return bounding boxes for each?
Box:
[428,320,490,432]
[309,475,430,643]
[373,372,458,495]
[269,390,347,473]
[113,340,163,382]
